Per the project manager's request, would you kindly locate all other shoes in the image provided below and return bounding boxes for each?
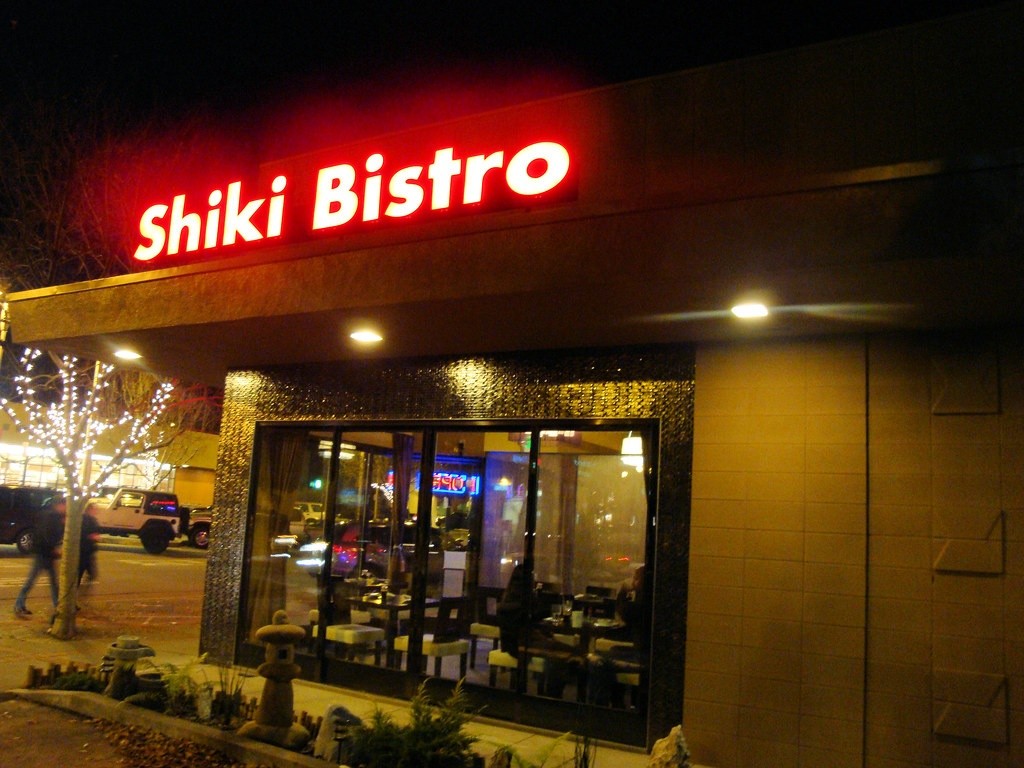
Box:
[15,607,32,614]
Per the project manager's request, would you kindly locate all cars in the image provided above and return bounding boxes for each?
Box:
[310,498,471,603]
[1,485,66,552]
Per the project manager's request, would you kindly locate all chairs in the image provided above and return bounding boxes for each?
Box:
[471,585,647,711]
[394,595,471,681]
[312,574,386,667]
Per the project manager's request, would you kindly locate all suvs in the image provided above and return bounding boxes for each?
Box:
[187,496,311,551]
[83,488,190,555]
[296,500,326,528]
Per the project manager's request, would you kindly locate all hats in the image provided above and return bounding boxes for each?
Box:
[634,565,644,587]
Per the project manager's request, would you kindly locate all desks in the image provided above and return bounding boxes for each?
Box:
[345,594,439,668]
[542,616,620,705]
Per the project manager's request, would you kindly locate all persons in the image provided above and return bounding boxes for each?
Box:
[589,565,644,708]
[76,503,100,594]
[14,496,67,618]
[496,564,570,698]
